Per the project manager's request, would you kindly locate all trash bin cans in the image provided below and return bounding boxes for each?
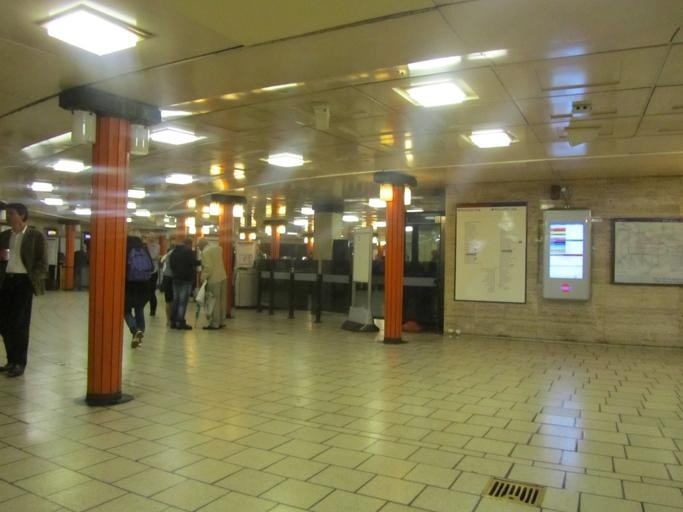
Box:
[233,266,259,308]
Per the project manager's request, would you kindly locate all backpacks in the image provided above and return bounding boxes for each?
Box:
[127,246,153,283]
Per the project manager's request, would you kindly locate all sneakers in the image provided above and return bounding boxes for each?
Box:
[0,361,24,376]
[171,322,191,329]
[132,330,144,348]
[203,325,225,329]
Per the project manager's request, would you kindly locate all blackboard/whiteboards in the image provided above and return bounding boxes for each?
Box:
[351,231,372,283]
[452,199,529,306]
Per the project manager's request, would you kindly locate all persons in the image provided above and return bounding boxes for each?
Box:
[196,238,228,330]
[0,203,45,376]
[139,229,179,327]
[124,231,150,348]
[168,237,201,329]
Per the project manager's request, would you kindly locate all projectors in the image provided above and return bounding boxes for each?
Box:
[571,103,592,118]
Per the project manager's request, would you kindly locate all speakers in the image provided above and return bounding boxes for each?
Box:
[551,184,560,200]
